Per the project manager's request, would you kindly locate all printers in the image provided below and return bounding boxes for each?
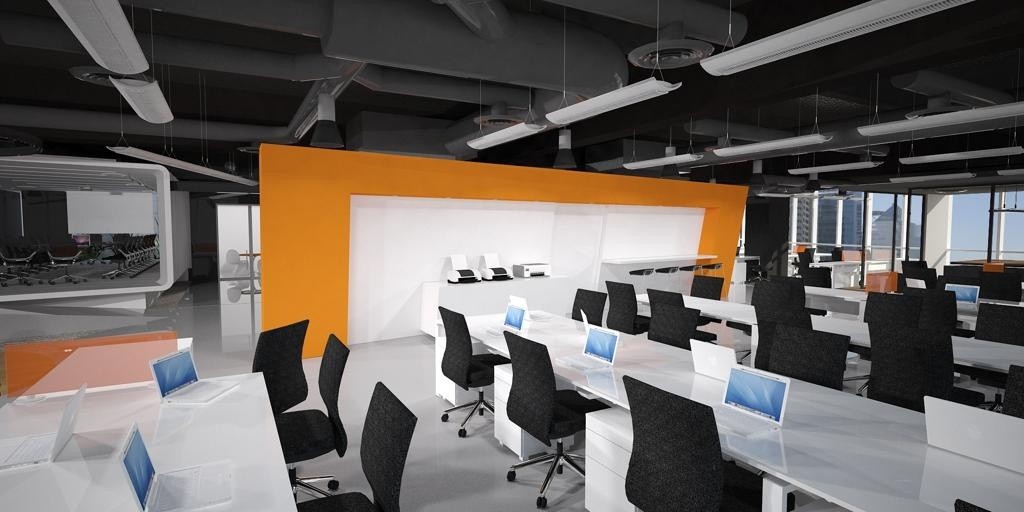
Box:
[447,254,551,283]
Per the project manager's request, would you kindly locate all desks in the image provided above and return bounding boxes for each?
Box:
[1,370,298,511]
[433,310,934,511]
[13,337,191,400]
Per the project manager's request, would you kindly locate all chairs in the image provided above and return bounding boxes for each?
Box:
[273,334,350,501]
[975,304,1024,411]
[251,318,309,412]
[862,291,984,407]
[420,244,1024,327]
[437,306,511,439]
[503,330,610,510]
[602,296,638,337]
[0,226,161,288]
[851,312,946,414]
[998,365,1024,419]
[750,274,813,371]
[769,323,852,392]
[622,374,796,512]
[647,302,701,350]
[647,288,717,343]
[296,381,418,511]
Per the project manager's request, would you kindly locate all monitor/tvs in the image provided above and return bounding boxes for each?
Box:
[73,235,90,247]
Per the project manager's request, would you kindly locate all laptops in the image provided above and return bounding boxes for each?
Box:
[944,284,979,315]
[0,382,88,470]
[688,338,791,439]
[482,295,554,338]
[149,347,238,404]
[555,309,620,374]
[118,421,203,512]
[923,393,1024,475]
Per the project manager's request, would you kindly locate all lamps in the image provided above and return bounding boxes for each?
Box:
[698,1,969,77]
[858,70,1023,138]
[623,111,703,170]
[713,87,833,158]
[898,116,1022,165]
[996,131,1023,175]
[552,130,579,170]
[310,84,344,149]
[889,135,976,183]
[106,1,176,124]
[103,66,260,189]
[546,4,682,126]
[466,79,546,150]
[48,1,150,75]
[788,90,884,175]
